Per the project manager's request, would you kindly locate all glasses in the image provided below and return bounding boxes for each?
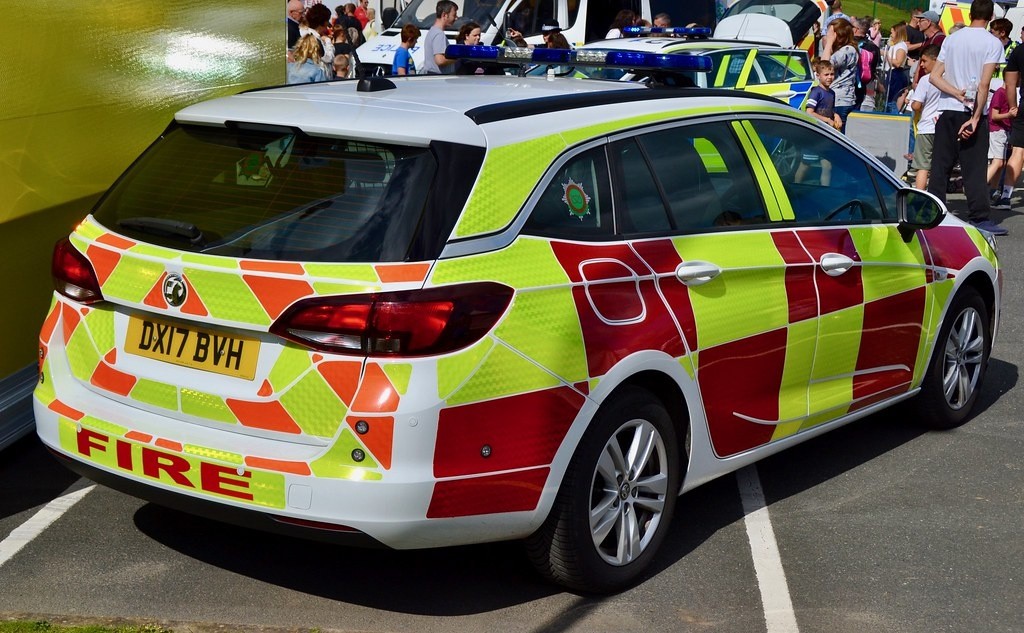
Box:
[364,2,368,3]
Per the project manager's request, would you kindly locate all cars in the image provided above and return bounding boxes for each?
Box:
[518,15,818,111]
[31,43,1001,600]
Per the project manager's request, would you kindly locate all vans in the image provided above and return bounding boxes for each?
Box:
[345,0,719,79]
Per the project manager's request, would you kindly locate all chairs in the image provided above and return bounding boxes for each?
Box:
[327,149,390,221]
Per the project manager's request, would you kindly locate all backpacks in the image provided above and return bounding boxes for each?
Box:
[859,47,873,83]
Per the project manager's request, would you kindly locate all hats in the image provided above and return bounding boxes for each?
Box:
[539,20,562,34]
[916,11,939,23]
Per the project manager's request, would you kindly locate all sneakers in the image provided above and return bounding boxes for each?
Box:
[990,196,1011,209]
[969,220,1009,234]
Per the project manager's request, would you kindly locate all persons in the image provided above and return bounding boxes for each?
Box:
[423,0,459,74]
[286,0,385,84]
[604,7,704,40]
[391,22,421,75]
[805,0,1024,235]
[455,19,576,79]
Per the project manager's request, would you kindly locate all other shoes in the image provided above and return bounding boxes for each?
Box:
[993,189,1001,199]
